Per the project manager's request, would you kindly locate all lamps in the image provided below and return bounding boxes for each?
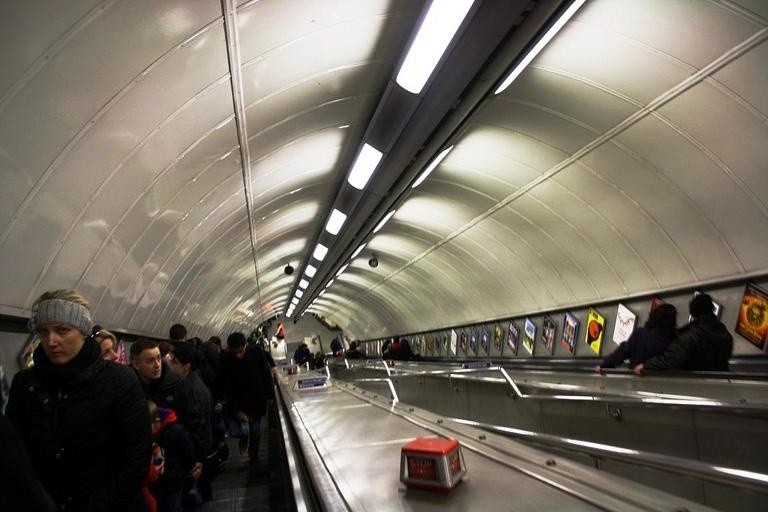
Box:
[284,263,295,275]
[368,254,379,268]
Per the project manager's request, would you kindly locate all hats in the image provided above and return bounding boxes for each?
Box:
[89,326,118,348]
[689,294,717,316]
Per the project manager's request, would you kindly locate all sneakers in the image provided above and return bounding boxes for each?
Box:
[239,434,248,455]
[219,446,229,461]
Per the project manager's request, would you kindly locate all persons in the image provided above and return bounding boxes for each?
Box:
[632,294,734,376]
[0,289,152,511]
[92,323,225,511]
[293,333,417,373]
[218,328,284,463]
[595,303,684,376]
